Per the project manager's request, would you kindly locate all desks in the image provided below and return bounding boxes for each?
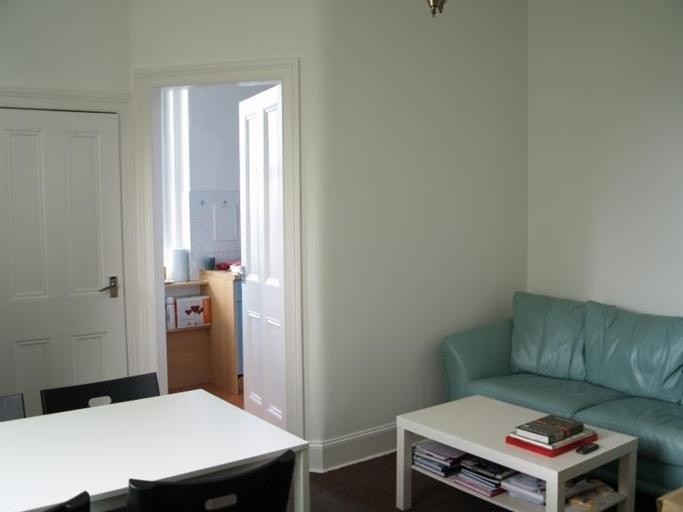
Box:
[0,387,312,512]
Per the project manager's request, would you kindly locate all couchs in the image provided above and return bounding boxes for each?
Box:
[433,289,683,493]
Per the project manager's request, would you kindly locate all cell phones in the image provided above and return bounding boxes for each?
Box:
[576,443,598,454]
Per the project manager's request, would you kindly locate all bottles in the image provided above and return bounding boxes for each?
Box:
[166,297,176,330]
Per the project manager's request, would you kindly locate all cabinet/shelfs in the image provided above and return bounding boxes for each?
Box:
[161,280,212,334]
[199,269,241,395]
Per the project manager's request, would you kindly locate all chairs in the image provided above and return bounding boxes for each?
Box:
[26,489,92,511]
[2,392,28,421]
[124,449,295,511]
[40,371,159,417]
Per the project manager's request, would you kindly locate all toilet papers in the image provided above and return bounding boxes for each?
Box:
[171,248,189,282]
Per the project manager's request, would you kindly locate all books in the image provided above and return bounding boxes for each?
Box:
[410,415,614,510]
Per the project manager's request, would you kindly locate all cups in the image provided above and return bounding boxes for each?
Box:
[204,256,215,271]
